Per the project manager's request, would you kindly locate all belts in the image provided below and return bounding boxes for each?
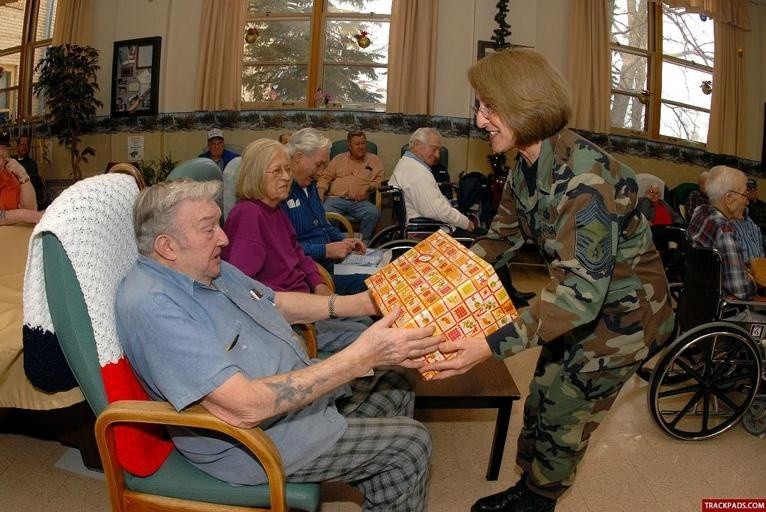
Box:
[722,305,750,319]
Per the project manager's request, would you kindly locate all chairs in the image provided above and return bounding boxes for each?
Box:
[0,138,700,512]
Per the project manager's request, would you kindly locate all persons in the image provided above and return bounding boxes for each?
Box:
[115,178,445,512]
[10,134,49,212]
[685,165,766,323]
[419,48,674,512]
[0,131,46,226]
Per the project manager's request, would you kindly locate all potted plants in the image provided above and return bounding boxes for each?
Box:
[30,41,104,205]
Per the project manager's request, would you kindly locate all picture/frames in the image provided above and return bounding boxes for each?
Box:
[473,38,537,125]
[110,35,163,118]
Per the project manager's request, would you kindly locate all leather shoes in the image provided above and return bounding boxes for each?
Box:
[509,290,536,300]
[470,472,557,512]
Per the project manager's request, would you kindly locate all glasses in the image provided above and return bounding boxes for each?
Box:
[265,167,290,176]
[471,105,496,119]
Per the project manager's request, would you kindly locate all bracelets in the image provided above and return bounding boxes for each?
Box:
[19,176,30,185]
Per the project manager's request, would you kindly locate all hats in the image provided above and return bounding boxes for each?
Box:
[207,128,225,142]
[129,95,138,103]
[747,179,757,189]
